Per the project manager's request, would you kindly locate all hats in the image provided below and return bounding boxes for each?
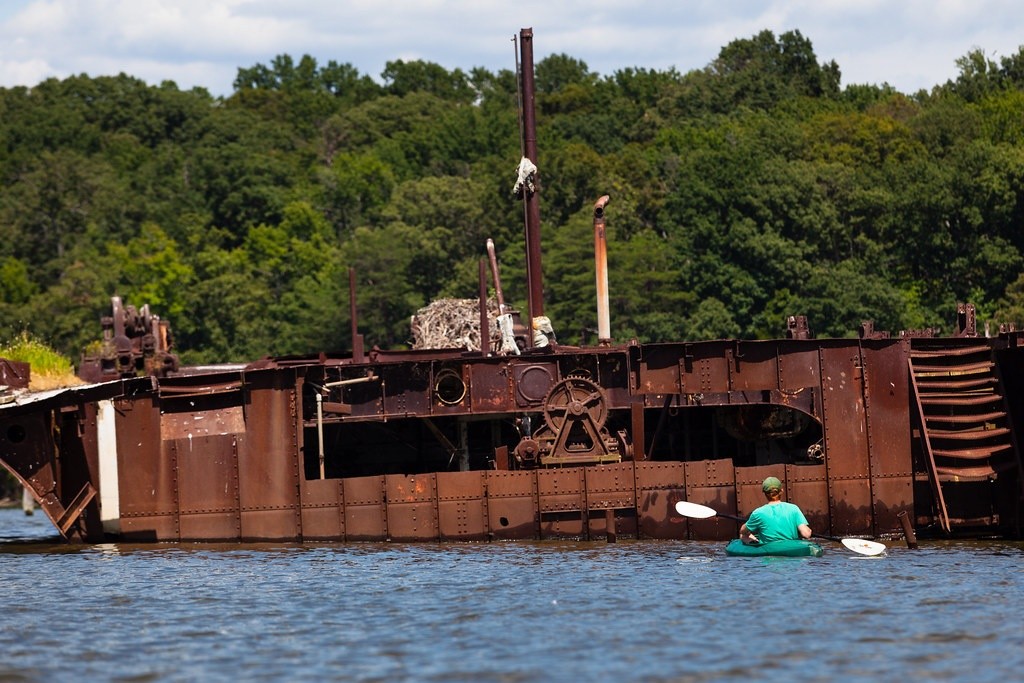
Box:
[761,476,782,492]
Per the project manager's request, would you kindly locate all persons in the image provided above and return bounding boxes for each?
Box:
[740,477,813,545]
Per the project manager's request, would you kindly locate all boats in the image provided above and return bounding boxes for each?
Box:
[726,538,824,558]
[4,29,1020,541]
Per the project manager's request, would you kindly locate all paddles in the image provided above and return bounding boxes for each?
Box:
[675,501,886,556]
